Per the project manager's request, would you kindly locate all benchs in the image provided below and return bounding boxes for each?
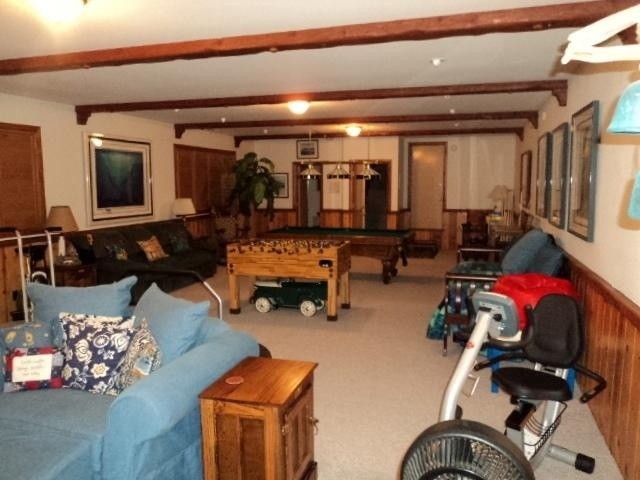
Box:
[441,228,564,357]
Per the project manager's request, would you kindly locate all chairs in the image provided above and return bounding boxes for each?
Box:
[462,209,489,259]
[0,304,272,480]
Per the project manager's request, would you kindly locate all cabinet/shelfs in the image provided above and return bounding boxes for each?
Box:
[199,355,319,480]
[42,267,96,290]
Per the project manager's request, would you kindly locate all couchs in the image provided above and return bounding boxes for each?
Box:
[77,219,219,303]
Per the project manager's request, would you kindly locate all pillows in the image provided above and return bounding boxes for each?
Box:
[105,226,192,262]
[0,277,211,401]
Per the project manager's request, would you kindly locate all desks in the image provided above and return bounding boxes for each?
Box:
[264,225,417,285]
[485,215,522,261]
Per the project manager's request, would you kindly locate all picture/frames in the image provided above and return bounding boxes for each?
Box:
[295,139,319,159]
[266,172,290,199]
[519,99,601,242]
[81,131,154,228]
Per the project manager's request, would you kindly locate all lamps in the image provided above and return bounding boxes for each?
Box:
[171,198,197,228]
[45,205,79,257]
[297,128,384,181]
